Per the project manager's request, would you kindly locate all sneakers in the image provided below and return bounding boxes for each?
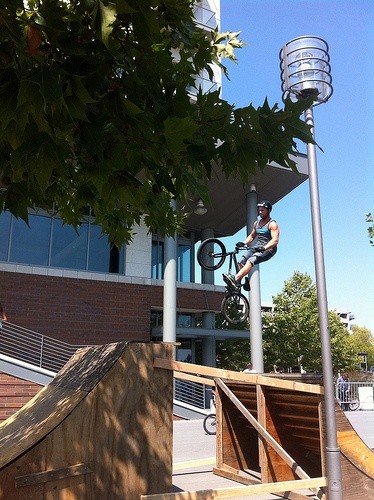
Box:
[223,273,238,289]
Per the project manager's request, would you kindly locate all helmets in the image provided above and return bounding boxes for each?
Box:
[257,200,273,212]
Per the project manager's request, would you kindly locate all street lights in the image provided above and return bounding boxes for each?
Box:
[278,35,343,499]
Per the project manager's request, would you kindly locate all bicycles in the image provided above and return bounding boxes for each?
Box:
[333,382,361,411]
[196,238,264,325]
[202,413,217,436]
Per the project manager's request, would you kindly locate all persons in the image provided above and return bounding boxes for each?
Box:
[222,200,280,290]
[338,373,351,411]
[243,362,257,373]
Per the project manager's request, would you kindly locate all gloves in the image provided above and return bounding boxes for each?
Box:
[236,242,245,247]
[255,245,265,253]
